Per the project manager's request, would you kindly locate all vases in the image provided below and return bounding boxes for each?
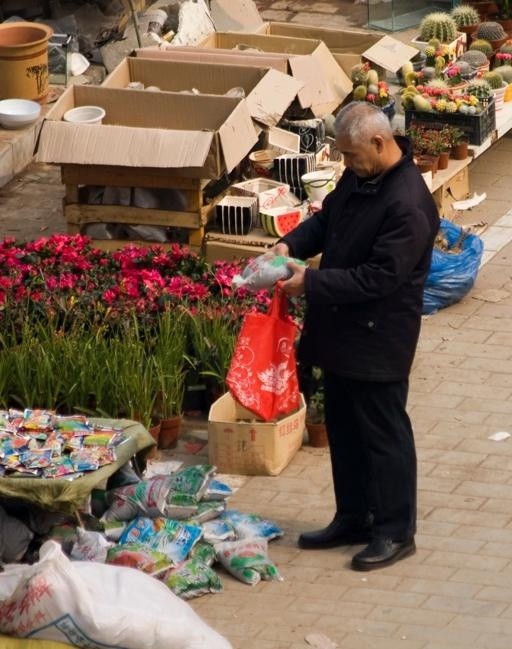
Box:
[155,414,184,449]
[149,421,162,449]
[1,22,53,106]
[311,423,328,449]
[212,151,338,238]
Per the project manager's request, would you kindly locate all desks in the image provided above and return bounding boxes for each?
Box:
[0,414,158,518]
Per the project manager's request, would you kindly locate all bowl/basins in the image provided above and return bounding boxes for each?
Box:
[63,105,105,124]
[0,98,41,130]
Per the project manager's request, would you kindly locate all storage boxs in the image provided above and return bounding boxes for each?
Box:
[208,390,307,477]
[129,44,336,111]
[99,56,304,131]
[255,21,421,80]
[33,84,260,190]
[197,33,355,98]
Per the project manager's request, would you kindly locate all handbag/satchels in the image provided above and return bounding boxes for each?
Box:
[224,281,303,423]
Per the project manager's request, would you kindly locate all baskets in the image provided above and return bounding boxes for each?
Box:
[405,97,495,145]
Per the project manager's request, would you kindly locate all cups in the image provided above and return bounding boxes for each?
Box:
[249,148,283,179]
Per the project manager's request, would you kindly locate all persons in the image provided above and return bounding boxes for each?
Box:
[265,99,441,571]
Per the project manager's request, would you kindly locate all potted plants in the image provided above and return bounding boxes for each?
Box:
[394,3,512,188]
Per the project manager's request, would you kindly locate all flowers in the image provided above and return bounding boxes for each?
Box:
[0,234,306,325]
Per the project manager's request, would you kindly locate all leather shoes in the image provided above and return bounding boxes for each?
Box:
[298,516,372,549]
[352,536,415,571]
